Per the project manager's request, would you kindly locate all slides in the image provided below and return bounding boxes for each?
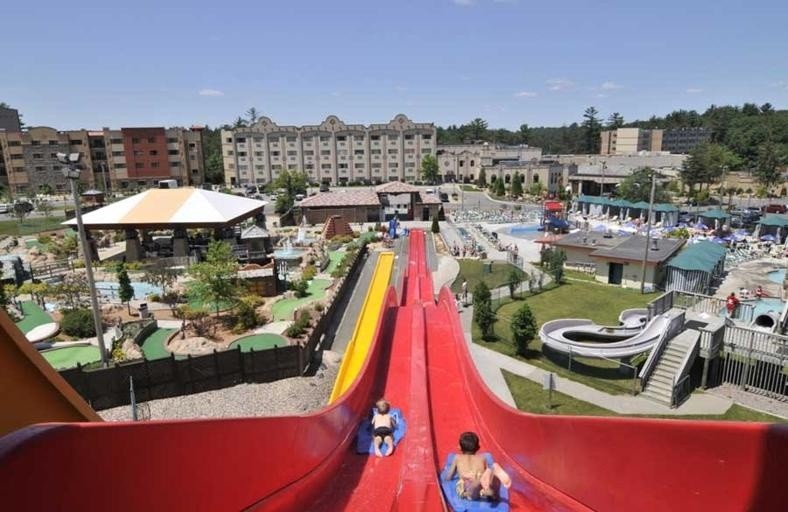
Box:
[0,228,784,511]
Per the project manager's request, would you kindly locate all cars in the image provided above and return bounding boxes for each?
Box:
[426,188,448,202]
[246,184,329,201]
[0,201,32,213]
[727,202,788,223]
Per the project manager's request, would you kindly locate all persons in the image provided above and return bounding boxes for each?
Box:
[444,432,513,502]
[365,400,396,459]
[754,285,763,299]
[458,278,469,304]
[454,294,460,312]
[446,233,520,263]
[725,292,739,319]
[748,246,759,256]
[140,239,161,259]
[307,242,315,257]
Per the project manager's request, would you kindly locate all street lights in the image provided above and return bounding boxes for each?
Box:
[599,159,607,197]
[54,151,109,366]
[638,173,661,295]
